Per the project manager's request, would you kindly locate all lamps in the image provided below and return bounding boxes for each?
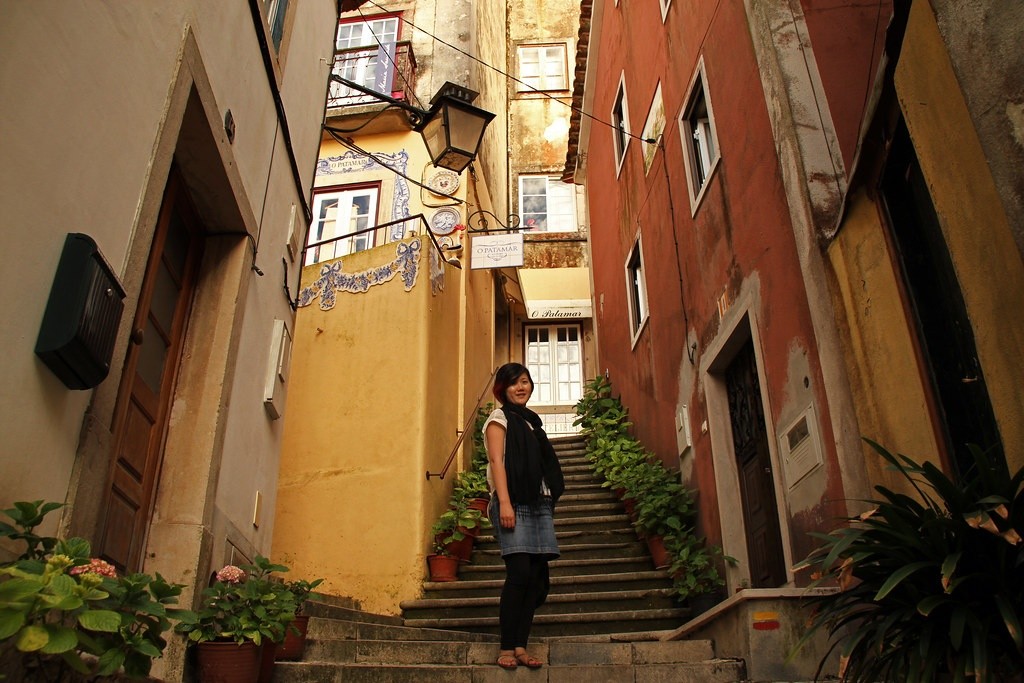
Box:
[330,72,497,177]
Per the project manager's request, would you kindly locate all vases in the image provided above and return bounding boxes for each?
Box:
[261,630,278,683]
[274,613,313,662]
[194,635,267,683]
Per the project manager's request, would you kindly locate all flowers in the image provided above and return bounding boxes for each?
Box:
[0,498,324,683]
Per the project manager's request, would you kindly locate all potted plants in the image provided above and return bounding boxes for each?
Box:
[425,405,500,585]
[571,376,741,607]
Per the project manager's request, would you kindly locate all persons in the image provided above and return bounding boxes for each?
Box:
[481,363,565,669]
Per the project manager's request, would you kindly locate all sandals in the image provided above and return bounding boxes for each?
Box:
[515,653,543,667]
[497,652,517,668]
